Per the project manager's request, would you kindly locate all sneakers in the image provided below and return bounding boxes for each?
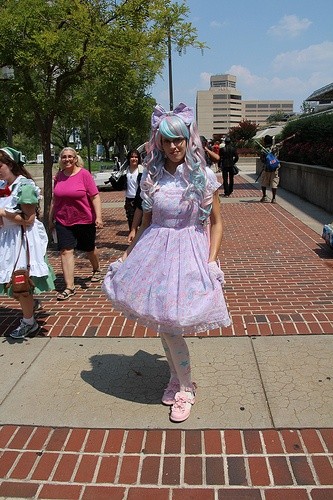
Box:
[260,196,267,203]
[9,319,38,338]
[271,198,277,203]
[15,299,41,317]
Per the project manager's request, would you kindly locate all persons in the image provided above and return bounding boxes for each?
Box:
[101,103,233,421]
[48,147,101,301]
[105,141,109,158]
[218,138,239,197]
[205,138,226,168]
[109,149,145,232]
[259,135,283,203]
[0,147,57,338]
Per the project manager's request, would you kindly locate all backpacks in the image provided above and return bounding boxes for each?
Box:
[265,153,280,172]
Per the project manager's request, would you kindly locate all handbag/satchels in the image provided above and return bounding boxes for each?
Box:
[205,150,214,166]
[6,270,35,299]
[233,164,239,175]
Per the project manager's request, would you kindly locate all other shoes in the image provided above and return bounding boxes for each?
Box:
[162,377,198,422]
[214,169,220,173]
[225,194,232,198]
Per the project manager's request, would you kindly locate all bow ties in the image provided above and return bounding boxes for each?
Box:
[0,185,11,198]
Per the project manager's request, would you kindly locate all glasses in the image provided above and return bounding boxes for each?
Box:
[162,137,185,147]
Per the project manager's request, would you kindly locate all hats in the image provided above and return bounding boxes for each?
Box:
[207,139,212,142]
[224,138,231,142]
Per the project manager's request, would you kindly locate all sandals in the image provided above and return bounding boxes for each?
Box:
[57,287,77,300]
[90,269,102,282]
